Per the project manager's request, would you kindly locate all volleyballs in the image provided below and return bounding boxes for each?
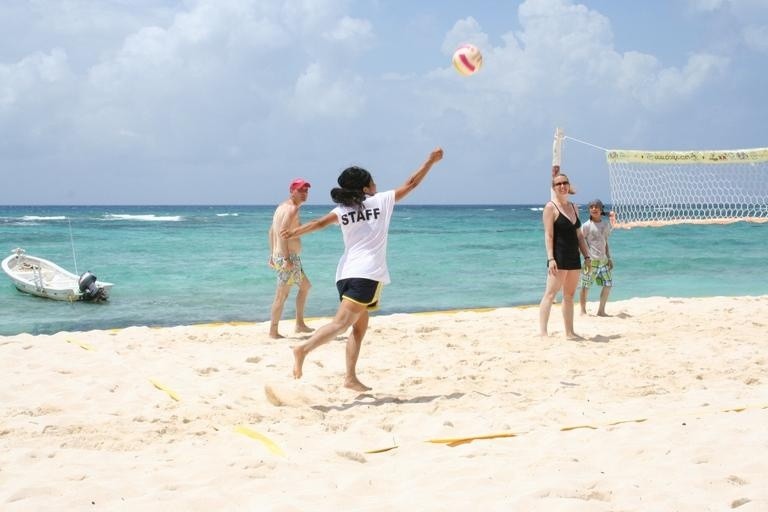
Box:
[452,43,483,74]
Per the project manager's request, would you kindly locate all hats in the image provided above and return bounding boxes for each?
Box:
[288,178,313,193]
[587,198,603,208]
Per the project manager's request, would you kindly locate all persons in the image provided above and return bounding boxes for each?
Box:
[268,178,317,339]
[577,198,615,319]
[536,173,593,340]
[278,147,443,392]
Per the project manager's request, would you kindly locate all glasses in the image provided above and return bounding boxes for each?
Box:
[554,180,570,186]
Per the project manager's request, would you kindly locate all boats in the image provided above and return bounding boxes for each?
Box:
[1,248,114,301]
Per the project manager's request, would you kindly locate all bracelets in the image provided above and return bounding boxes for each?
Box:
[584,256,590,260]
[607,256,612,260]
[547,257,554,262]
